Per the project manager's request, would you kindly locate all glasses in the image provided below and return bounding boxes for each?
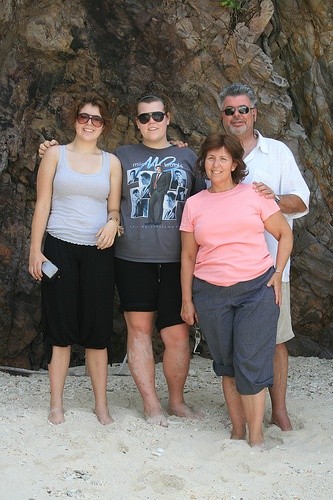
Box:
[222,105,254,115]
[137,112,165,124]
[77,113,104,127]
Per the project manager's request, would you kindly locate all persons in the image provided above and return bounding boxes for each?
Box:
[28,94,124,424]
[39,90,208,428]
[178,133,294,446]
[168,83,310,432]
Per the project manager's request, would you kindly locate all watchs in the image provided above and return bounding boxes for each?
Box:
[275,194,281,204]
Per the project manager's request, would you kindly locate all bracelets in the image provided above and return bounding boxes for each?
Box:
[108,217,124,238]
[275,269,282,274]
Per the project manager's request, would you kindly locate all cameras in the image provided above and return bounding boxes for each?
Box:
[35,261,63,282]
